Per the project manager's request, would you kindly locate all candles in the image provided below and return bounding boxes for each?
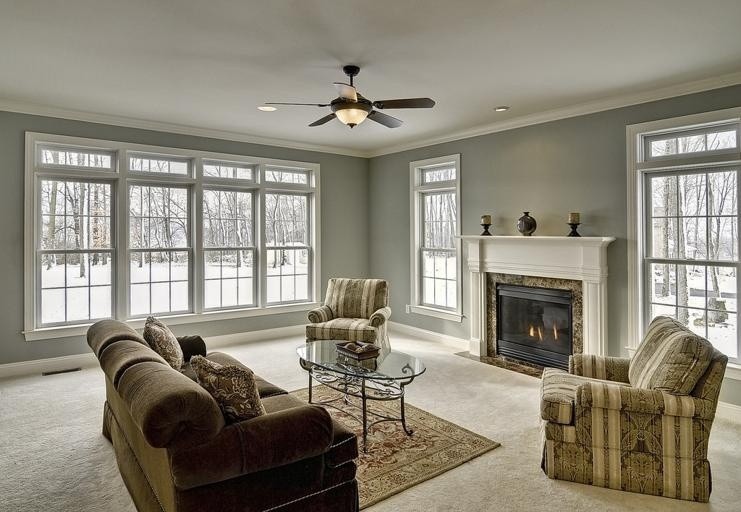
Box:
[481,214,491,224]
[569,213,579,223]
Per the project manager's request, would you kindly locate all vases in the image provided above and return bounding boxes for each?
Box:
[517,212,537,236]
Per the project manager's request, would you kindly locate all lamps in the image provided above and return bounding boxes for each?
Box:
[330,102,372,129]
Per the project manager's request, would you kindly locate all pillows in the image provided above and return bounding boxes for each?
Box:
[143,315,185,374]
[190,355,267,419]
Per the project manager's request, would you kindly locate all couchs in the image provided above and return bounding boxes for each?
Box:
[87,320,359,512]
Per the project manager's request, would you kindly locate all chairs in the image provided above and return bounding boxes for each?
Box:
[306,278,391,369]
[539,316,728,502]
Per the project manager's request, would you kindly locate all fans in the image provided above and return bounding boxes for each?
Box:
[265,65,435,127]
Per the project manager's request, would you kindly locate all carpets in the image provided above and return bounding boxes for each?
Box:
[289,384,501,512]
